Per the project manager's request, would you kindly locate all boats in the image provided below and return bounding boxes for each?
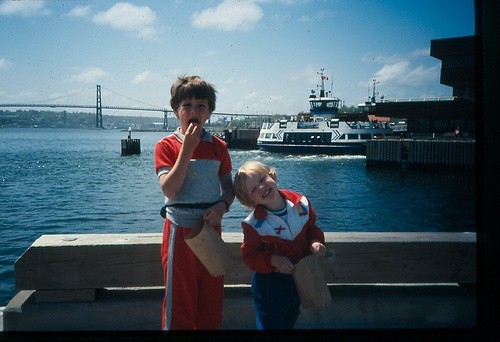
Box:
[256,67,407,156]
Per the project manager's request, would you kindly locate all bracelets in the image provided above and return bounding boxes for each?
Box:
[220,200,230,213]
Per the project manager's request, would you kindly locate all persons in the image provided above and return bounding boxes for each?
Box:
[233,161,327,331]
[155,75,236,332]
